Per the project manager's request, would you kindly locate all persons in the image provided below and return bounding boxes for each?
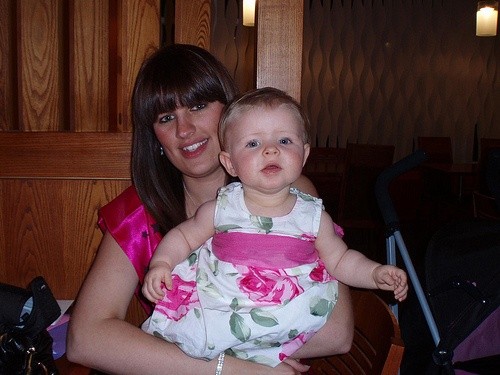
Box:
[140,86,407,367]
[66,43,353,375]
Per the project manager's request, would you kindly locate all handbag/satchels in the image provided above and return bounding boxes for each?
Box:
[0,327,58,375]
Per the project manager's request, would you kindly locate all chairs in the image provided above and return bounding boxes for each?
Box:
[298,286,404,375]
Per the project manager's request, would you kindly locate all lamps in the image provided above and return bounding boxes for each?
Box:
[242,0,257,26]
[476,1,498,36]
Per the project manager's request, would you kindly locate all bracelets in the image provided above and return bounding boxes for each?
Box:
[215,353,225,375]
